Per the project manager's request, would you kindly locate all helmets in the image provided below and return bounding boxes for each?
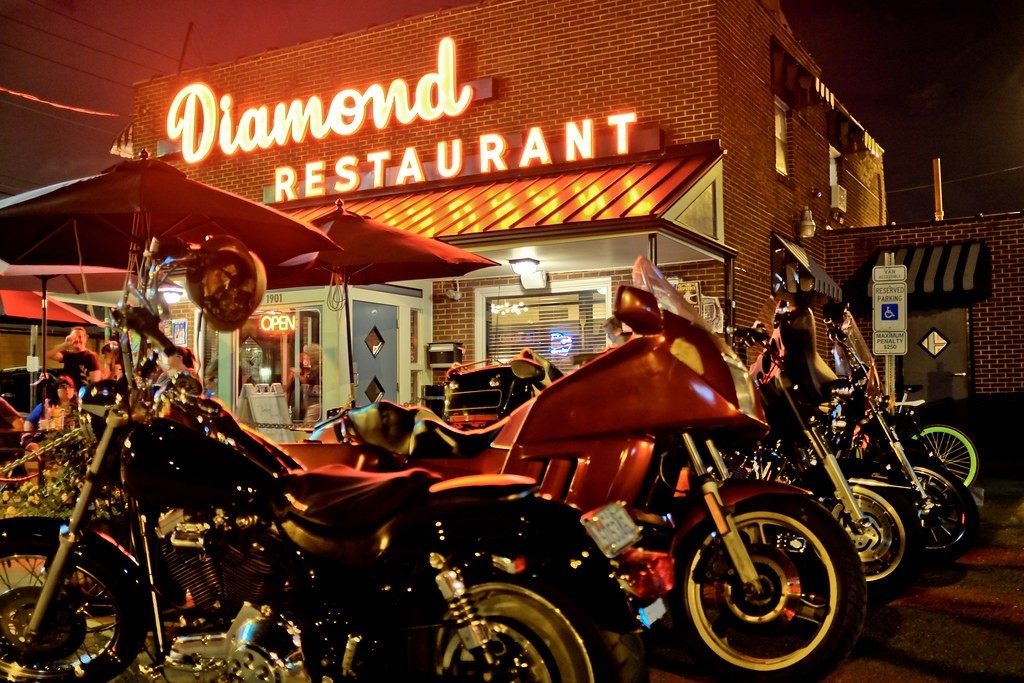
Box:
[186,234,268,332]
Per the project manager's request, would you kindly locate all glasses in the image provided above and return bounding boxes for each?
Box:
[58,383,74,389]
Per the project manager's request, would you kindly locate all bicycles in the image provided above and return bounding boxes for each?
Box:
[887,383,979,489]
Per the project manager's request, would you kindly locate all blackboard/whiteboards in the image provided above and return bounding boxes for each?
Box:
[237,382,295,441]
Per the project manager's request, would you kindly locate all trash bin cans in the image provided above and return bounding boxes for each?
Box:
[425,384,444,417]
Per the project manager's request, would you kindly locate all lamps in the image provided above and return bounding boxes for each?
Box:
[798,273,813,291]
[798,208,816,239]
[520,271,548,289]
[509,258,539,275]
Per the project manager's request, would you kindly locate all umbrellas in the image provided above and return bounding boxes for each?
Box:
[0,149,346,357]
[0,264,182,396]
[258,200,502,411]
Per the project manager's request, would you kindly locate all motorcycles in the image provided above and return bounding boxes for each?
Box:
[442,253,981,585]
[0,233,649,683]
[300,257,868,683]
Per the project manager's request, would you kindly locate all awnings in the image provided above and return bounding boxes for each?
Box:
[867,240,985,296]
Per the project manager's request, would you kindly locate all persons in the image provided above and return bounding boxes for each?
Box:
[48,326,101,392]
[21,375,77,485]
[101,343,123,379]
[286,343,321,422]
